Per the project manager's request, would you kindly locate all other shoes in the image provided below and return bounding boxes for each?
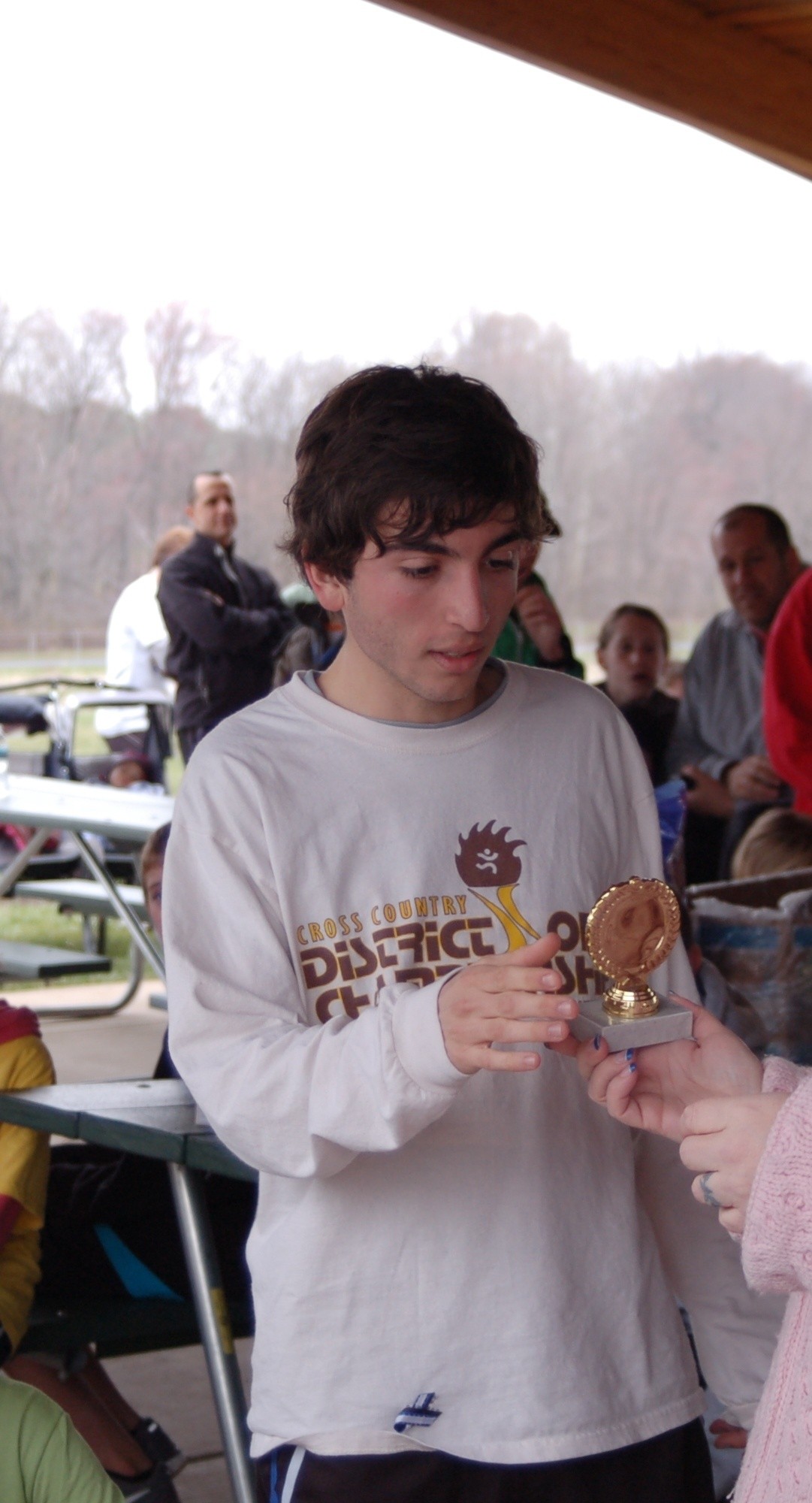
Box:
[107,1454,182,1503]
[128,1418,181,1465]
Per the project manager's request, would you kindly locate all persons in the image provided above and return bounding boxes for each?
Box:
[162,360,707,1503]
[0,467,812,1503]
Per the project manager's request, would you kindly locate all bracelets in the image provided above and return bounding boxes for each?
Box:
[681,772,697,790]
[720,759,738,788]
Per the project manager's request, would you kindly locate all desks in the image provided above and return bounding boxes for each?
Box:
[0,776,172,988]
[2,1078,259,1503]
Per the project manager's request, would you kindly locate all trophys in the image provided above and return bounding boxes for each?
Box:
[571,875,692,1055]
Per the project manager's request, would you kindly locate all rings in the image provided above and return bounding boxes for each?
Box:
[699,1174,721,1207]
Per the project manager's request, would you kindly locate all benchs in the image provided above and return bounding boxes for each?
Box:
[0,876,152,1020]
